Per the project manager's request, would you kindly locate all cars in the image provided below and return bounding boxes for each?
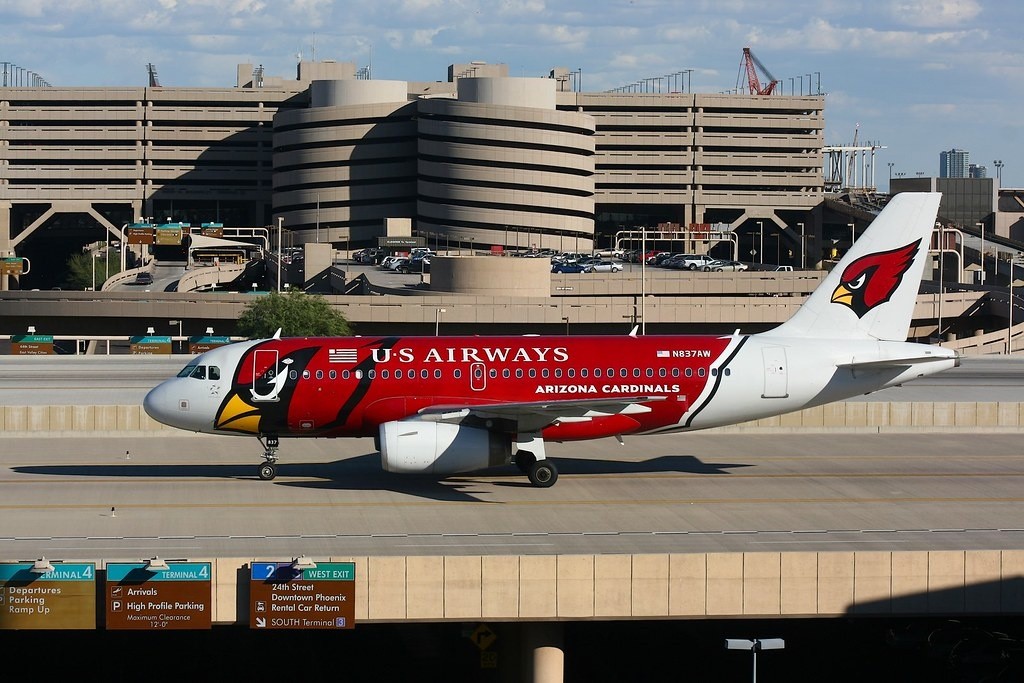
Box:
[506,248,792,279]
[136,271,153,285]
[353,248,435,273]
[276,248,304,265]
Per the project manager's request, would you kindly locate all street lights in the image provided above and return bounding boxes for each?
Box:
[0,62,52,87]
[169,320,182,350]
[994,160,1004,188]
[339,236,349,272]
[504,225,739,273]
[888,163,924,179]
[976,222,997,285]
[725,638,785,683]
[719,72,821,96]
[747,221,854,271]
[412,230,474,256]
[265,225,295,264]
[436,308,571,336]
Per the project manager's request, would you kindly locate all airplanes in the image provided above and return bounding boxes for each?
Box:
[143,193,961,486]
